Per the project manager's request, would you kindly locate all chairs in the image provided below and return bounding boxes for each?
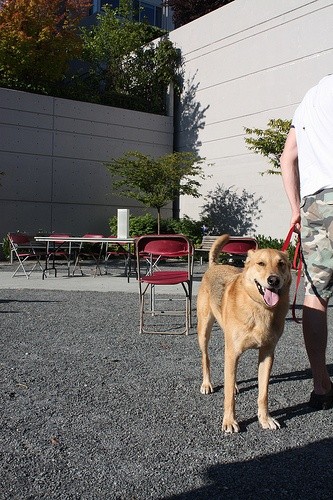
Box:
[135,234,192,336]
[8,232,142,280]
[221,238,259,255]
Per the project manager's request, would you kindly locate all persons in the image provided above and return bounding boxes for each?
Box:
[280,72,333,409]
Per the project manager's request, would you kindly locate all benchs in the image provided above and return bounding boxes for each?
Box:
[196,234,255,265]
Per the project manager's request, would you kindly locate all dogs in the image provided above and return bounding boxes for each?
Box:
[194,231,293,434]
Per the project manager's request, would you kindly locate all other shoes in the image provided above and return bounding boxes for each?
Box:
[308,385,333,410]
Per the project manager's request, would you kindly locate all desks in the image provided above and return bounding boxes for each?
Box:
[36,235,134,274]
